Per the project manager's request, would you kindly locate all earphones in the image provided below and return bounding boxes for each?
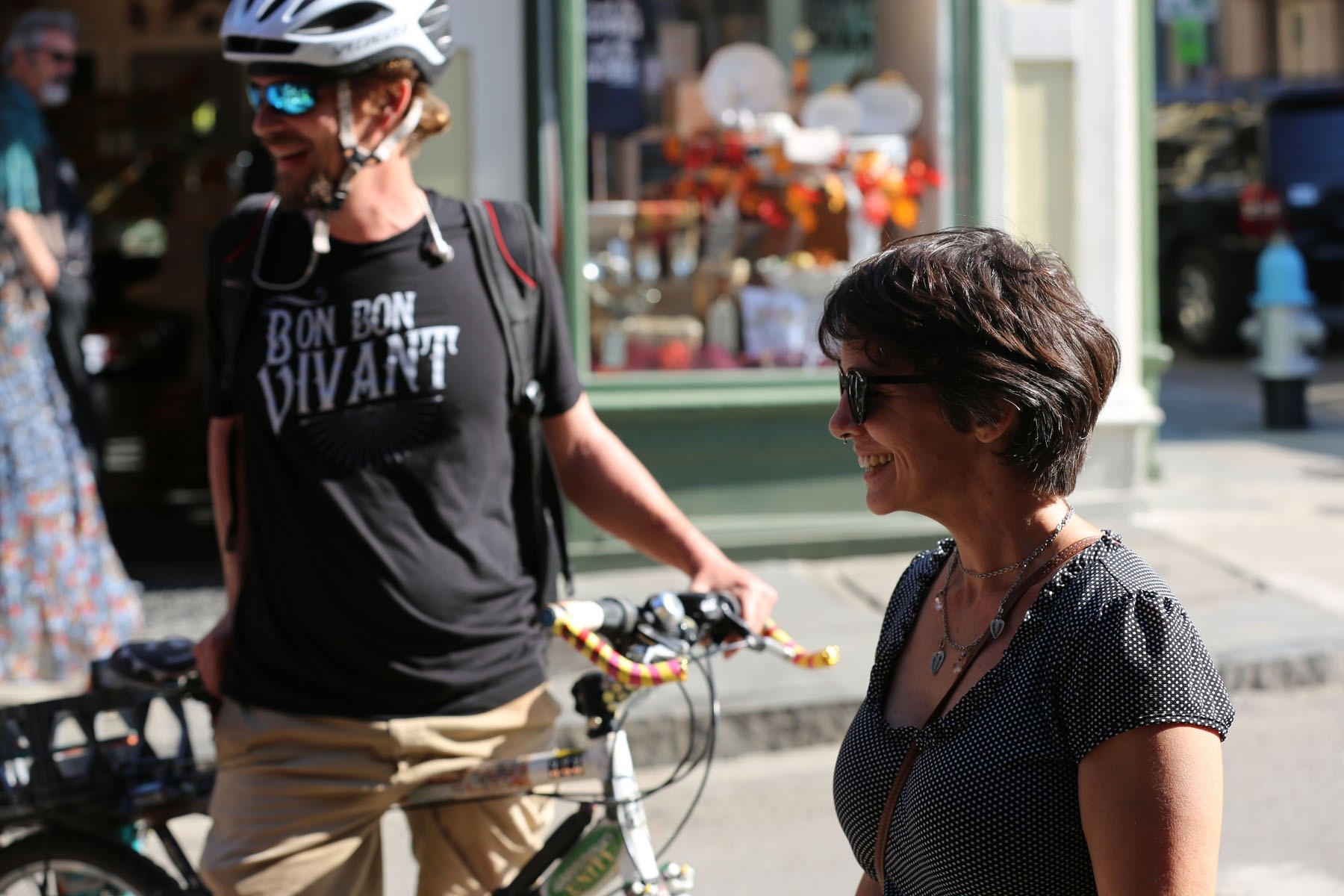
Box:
[431,238,454,263]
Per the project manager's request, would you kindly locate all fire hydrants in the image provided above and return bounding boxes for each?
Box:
[1238,231,1330,435]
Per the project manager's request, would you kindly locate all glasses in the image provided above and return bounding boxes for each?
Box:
[34,46,78,64]
[243,63,380,117]
[838,359,965,426]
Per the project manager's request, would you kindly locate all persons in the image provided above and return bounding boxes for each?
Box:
[194,1,780,894]
[815,227,1236,894]
[0,0,146,682]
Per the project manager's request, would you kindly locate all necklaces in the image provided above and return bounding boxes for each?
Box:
[929,498,1074,677]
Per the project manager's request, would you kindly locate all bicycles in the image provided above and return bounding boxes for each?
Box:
[1,591,837,896]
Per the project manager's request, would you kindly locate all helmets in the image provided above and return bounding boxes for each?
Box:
[218,0,452,82]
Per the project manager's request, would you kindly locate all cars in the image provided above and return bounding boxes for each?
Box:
[1158,78,1344,350]
